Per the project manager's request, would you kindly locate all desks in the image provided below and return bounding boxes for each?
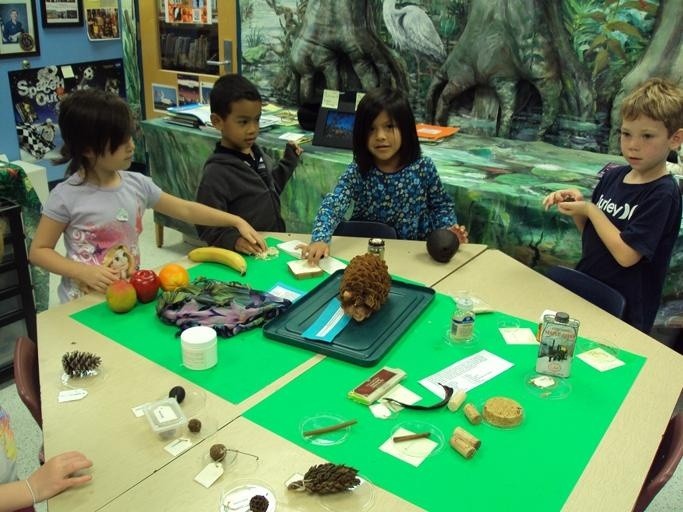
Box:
[36,231,683,512]
[139,104,683,299]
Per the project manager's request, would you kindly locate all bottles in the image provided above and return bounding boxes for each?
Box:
[536,313,581,379]
[367,238,386,260]
[449,297,476,342]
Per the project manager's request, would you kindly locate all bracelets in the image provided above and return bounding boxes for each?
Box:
[25,479,35,505]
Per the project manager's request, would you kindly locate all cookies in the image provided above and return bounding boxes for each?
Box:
[483,397,522,427]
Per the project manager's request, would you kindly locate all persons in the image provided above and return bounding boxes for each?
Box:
[542,78,683,335]
[195,75,304,257]
[3,9,25,43]
[294,87,469,267]
[0,408,92,512]
[27,89,267,304]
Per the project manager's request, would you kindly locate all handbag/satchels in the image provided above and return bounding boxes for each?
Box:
[157,276,293,340]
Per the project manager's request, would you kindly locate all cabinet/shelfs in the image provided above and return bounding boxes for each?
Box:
[0,194,36,383]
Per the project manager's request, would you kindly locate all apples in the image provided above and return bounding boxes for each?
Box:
[105,280,137,312]
[130,268,158,304]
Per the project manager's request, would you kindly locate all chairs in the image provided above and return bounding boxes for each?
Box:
[544,264,627,319]
[633,415,683,512]
[332,220,398,239]
[14,336,44,466]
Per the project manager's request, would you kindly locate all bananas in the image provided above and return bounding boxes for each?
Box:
[187,247,246,276]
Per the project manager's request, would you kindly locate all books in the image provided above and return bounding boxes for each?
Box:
[163,102,212,128]
[259,115,281,132]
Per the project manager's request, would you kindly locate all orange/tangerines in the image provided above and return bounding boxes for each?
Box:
[158,263,188,292]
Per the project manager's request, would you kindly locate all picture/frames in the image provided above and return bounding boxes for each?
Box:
[0,0,40,59]
[312,101,357,150]
[40,0,84,28]
